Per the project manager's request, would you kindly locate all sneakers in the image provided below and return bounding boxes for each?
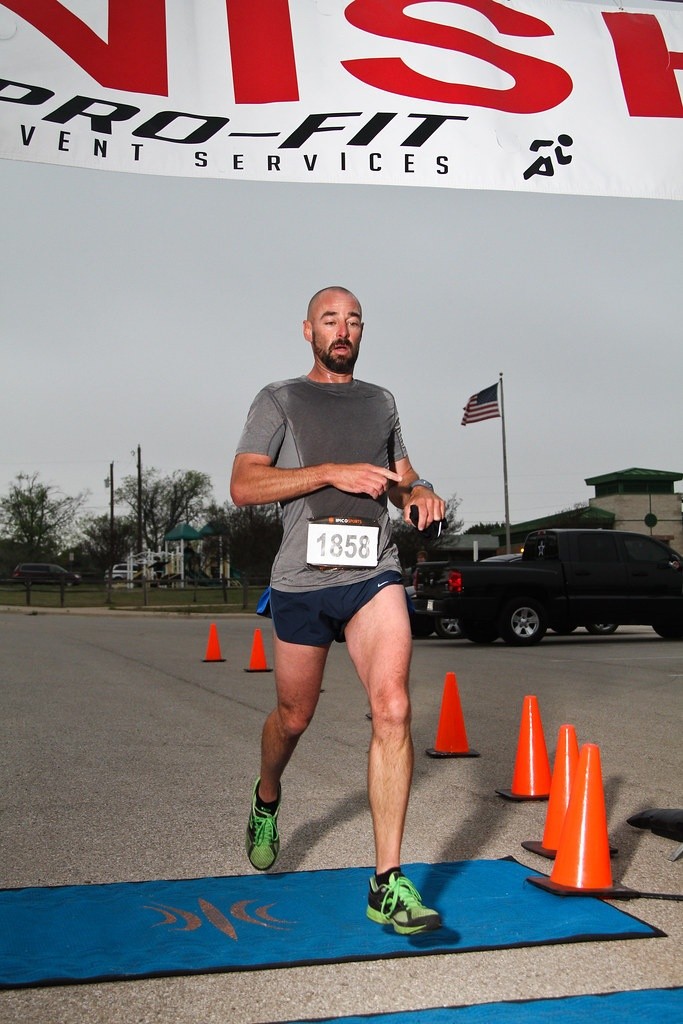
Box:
[245,776,282,870]
[366,869,442,934]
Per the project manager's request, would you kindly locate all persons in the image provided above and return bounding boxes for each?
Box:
[148,540,197,583]
[230,287,446,935]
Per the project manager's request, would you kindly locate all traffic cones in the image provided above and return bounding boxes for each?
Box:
[424,672,482,758]
[494,696,552,802]
[520,742,639,897]
[520,724,620,860]
[242,628,274,674]
[200,623,228,663]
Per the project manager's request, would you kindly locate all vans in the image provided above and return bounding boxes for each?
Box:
[13,563,81,589]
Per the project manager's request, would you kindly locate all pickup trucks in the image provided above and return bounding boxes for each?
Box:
[410,526,682,648]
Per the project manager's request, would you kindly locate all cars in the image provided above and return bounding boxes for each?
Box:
[104,563,157,587]
[402,572,465,640]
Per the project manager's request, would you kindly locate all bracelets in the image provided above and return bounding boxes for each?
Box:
[407,478,434,494]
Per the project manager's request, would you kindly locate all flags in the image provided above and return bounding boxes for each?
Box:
[460,382,502,426]
